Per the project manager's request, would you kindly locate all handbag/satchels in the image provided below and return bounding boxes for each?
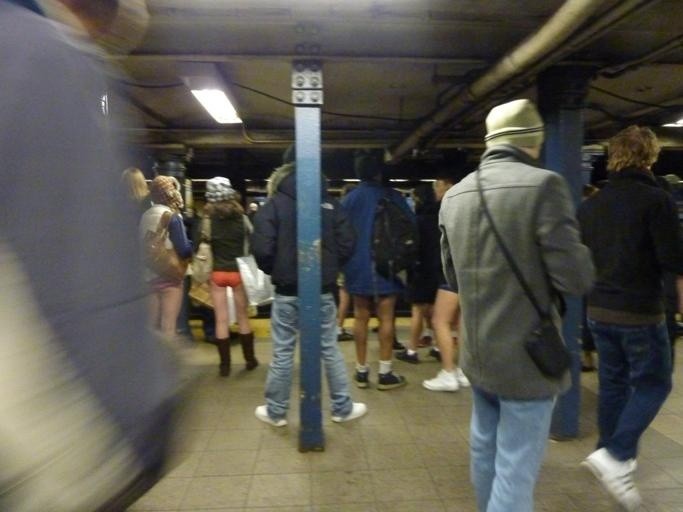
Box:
[139,207,193,287]
[521,312,576,380]
[190,240,215,285]
[235,250,277,308]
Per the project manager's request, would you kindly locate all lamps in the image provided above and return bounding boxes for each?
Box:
[174,60,244,123]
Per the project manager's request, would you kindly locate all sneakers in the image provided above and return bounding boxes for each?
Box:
[417,336,432,348]
[376,370,407,391]
[421,368,460,392]
[352,368,369,388]
[336,329,354,342]
[580,445,644,512]
[330,402,368,423]
[394,349,418,364]
[429,348,442,362]
[456,367,472,387]
[254,404,288,427]
[392,333,405,350]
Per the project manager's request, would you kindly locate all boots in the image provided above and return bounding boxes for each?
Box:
[238,331,258,370]
[214,337,231,377]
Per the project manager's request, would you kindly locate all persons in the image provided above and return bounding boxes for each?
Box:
[251,144,368,428]
[438,98,597,512]
[123,155,682,391]
[576,124,682,511]
[1,1,202,512]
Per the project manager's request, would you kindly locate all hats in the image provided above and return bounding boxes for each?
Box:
[150,174,185,209]
[203,176,237,203]
[483,96,546,149]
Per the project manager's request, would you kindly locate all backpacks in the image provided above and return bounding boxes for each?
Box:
[361,180,425,291]
